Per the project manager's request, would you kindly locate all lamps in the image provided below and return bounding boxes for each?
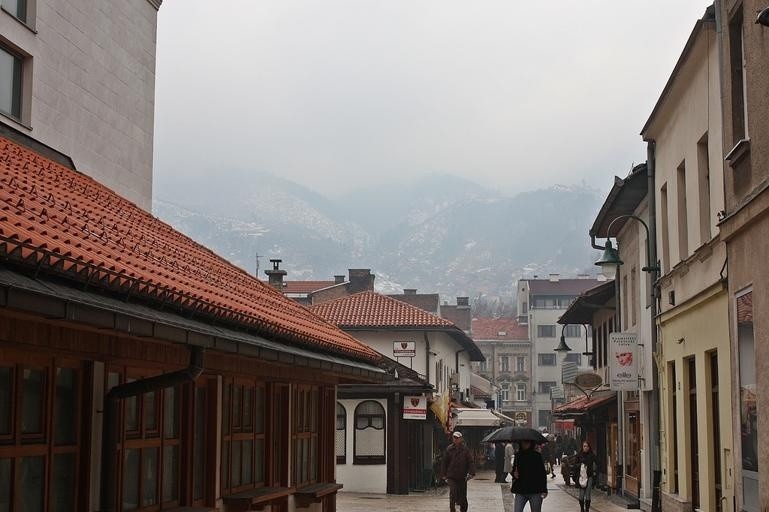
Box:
[553,324,594,356]
[594,216,661,278]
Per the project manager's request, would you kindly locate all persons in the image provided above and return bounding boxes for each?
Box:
[453,438,467,505]
[511,440,548,512]
[481,425,577,484]
[441,432,473,512]
[615,352,632,366]
[573,441,598,512]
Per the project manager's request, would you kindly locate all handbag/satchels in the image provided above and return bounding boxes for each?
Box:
[579,463,588,488]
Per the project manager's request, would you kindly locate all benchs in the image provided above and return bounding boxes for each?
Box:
[222,482,345,511]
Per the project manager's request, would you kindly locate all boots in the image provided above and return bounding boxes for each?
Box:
[579,498,591,512]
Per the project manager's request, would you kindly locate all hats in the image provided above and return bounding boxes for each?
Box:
[453,431,463,438]
